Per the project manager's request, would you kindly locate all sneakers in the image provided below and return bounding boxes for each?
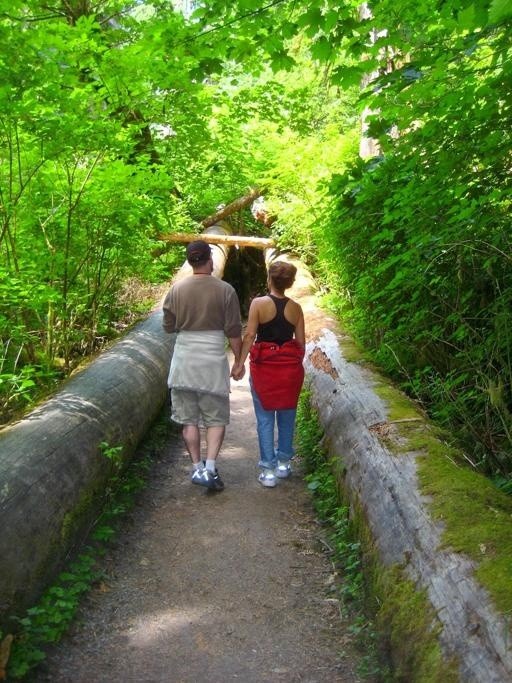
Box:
[275,461,292,479]
[257,469,276,488]
[191,460,224,491]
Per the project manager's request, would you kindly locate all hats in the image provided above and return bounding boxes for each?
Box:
[186,240,213,259]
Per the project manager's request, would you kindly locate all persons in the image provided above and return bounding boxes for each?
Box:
[163,240,245,492]
[233,262,305,487]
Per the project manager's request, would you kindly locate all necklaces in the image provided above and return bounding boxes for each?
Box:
[194,272,209,274]
